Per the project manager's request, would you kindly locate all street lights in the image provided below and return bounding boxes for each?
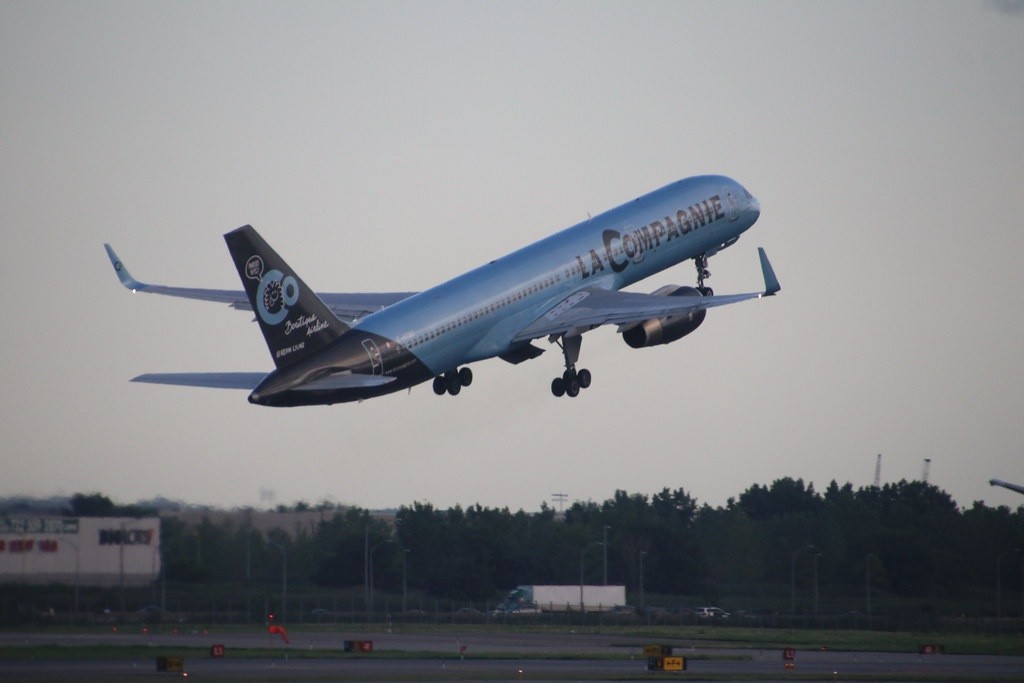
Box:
[369,540,393,602]
[640,550,647,609]
[603,525,613,586]
[402,548,411,621]
[579,541,603,621]
[363,518,404,619]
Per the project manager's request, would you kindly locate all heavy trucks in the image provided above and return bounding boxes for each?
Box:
[496,584,626,616]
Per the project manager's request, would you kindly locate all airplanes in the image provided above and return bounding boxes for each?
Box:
[101,173,781,407]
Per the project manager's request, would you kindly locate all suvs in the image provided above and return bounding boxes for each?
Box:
[697,607,730,619]
